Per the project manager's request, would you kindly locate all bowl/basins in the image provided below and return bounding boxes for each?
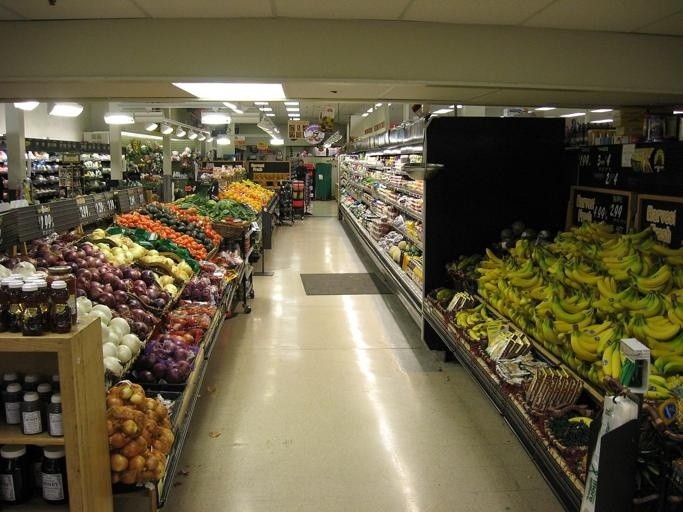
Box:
[403,163,444,180]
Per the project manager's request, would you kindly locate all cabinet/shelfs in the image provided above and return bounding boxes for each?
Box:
[339,117,563,351]
[288,120,309,140]
[421,118,682,512]
[0,135,254,512]
[170,144,313,276]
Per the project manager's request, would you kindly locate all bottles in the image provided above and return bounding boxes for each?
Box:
[0,263,77,337]
[0,442,70,508]
[3,371,65,438]
[564,118,616,146]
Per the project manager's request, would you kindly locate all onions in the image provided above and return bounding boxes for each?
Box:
[0,232,224,383]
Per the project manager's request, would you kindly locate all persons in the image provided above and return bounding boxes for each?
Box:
[296,159,308,181]
[222,166,231,173]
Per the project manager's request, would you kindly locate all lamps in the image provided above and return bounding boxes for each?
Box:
[145,118,213,143]
[13,101,40,111]
[201,112,231,125]
[104,112,135,125]
[47,101,83,117]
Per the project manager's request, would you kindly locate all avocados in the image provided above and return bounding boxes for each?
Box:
[139,203,214,252]
